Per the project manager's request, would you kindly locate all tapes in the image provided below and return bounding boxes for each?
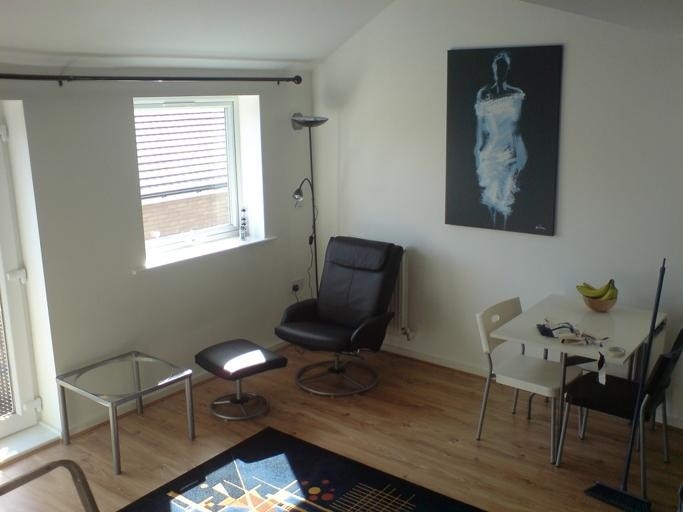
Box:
[606,345,626,358]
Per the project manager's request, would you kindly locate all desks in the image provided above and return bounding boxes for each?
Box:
[489,293,667,465]
[116,427,489,512]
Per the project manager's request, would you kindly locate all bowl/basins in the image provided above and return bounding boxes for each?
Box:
[583,294,618,313]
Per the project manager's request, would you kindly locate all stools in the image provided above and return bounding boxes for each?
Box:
[196,338,287,420]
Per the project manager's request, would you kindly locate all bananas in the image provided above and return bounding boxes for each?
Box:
[576,279,617,301]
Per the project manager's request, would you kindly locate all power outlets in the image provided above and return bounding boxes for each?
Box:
[290,278,305,297]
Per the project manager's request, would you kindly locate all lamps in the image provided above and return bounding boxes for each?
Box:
[291,112,330,301]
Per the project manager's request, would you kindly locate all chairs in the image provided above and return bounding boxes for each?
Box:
[554,329,683,500]
[274,236,405,396]
[476,296,584,465]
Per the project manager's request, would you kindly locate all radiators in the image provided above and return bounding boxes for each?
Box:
[386,248,412,341]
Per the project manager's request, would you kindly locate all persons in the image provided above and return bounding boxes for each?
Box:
[473,51,527,230]
